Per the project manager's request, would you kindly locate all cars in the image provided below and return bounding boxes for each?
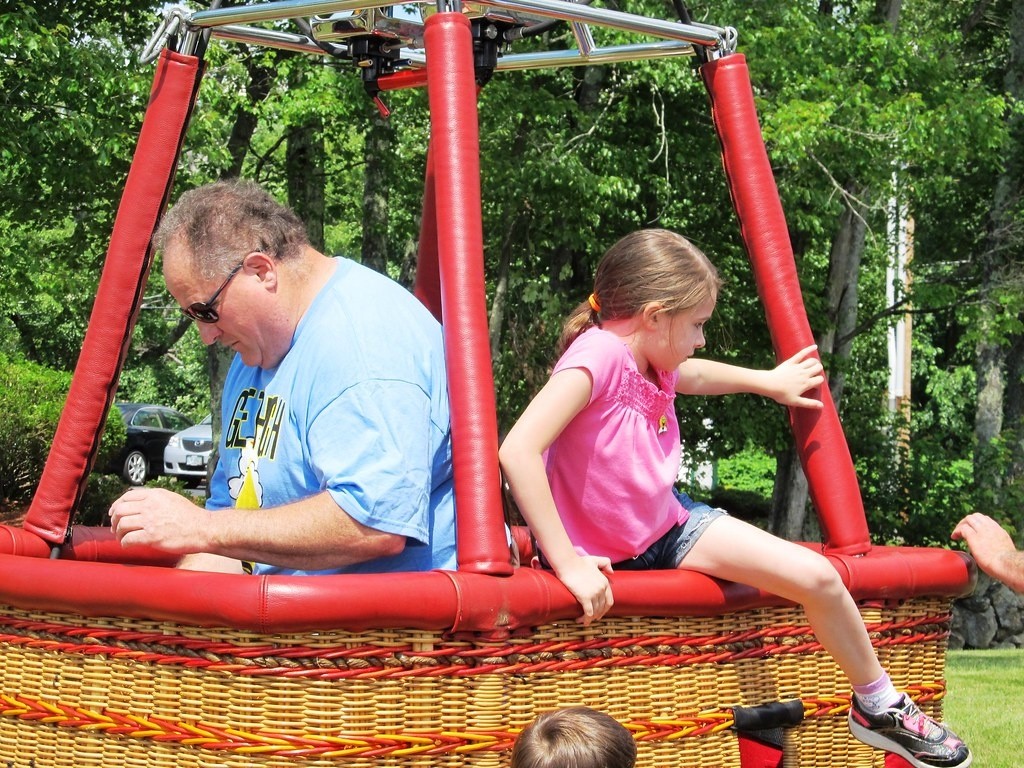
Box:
[100,403,196,487]
[162,412,214,489]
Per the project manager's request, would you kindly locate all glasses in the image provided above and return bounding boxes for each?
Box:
[178,252,262,324]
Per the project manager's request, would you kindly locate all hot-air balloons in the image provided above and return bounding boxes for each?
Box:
[1,0,981,767]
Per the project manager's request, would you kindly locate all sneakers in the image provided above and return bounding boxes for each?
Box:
[847,688,972,768]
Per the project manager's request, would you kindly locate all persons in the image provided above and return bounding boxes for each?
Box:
[109,177,457,576]
[498,229,1024,768]
